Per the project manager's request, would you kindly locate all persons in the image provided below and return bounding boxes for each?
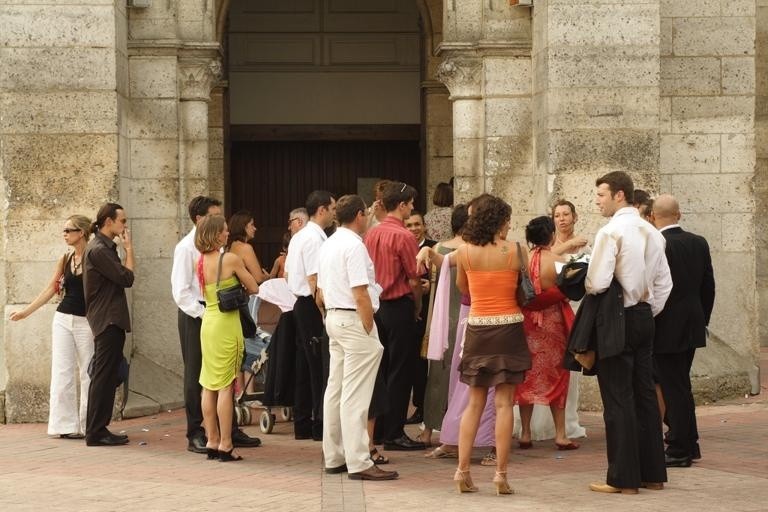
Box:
[9,214,95,439]
[82,202,134,447]
[171,171,715,494]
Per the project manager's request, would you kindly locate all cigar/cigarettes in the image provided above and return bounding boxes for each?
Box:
[125,230,132,232]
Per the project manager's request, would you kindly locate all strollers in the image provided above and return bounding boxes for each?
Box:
[234,278,299,435]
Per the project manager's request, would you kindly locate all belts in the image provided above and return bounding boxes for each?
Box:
[327,307,357,313]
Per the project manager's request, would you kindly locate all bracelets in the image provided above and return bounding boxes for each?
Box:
[123,247,132,250]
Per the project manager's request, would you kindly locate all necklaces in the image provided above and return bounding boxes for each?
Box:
[73,254,83,275]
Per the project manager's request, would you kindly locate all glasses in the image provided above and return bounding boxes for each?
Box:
[287,216,299,225]
[63,226,82,233]
[400,182,407,194]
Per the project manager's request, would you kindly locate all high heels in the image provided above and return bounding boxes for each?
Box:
[452,466,480,496]
[492,469,514,497]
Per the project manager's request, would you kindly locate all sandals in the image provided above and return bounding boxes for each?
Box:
[424,446,457,459]
[481,451,498,467]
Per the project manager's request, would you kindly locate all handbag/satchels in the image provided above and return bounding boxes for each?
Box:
[53,247,72,296]
[216,283,249,313]
[513,267,537,310]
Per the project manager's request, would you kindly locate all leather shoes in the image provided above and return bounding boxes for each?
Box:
[556,439,580,450]
[87,433,131,446]
[184,436,210,454]
[519,440,535,450]
[405,406,424,424]
[367,448,392,463]
[217,447,243,461]
[662,455,692,468]
[640,480,663,490]
[59,433,85,439]
[587,479,641,494]
[690,442,703,460]
[230,430,263,447]
[348,463,402,481]
[383,431,425,451]
[204,447,218,460]
[324,463,348,476]
